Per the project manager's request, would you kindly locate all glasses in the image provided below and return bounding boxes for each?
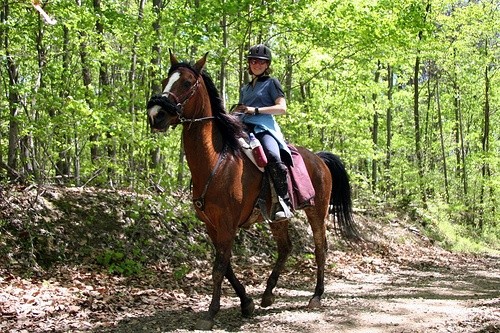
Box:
[249,58,268,64]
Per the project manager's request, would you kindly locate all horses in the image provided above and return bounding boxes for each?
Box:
[145,47,363,318]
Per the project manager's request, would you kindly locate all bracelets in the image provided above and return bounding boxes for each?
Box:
[255,106,258,113]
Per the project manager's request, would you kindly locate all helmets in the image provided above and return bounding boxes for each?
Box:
[247,43,271,61]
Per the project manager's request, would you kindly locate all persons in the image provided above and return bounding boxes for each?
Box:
[228,43,294,220]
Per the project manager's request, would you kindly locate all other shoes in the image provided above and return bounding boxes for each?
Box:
[275,202,297,220]
